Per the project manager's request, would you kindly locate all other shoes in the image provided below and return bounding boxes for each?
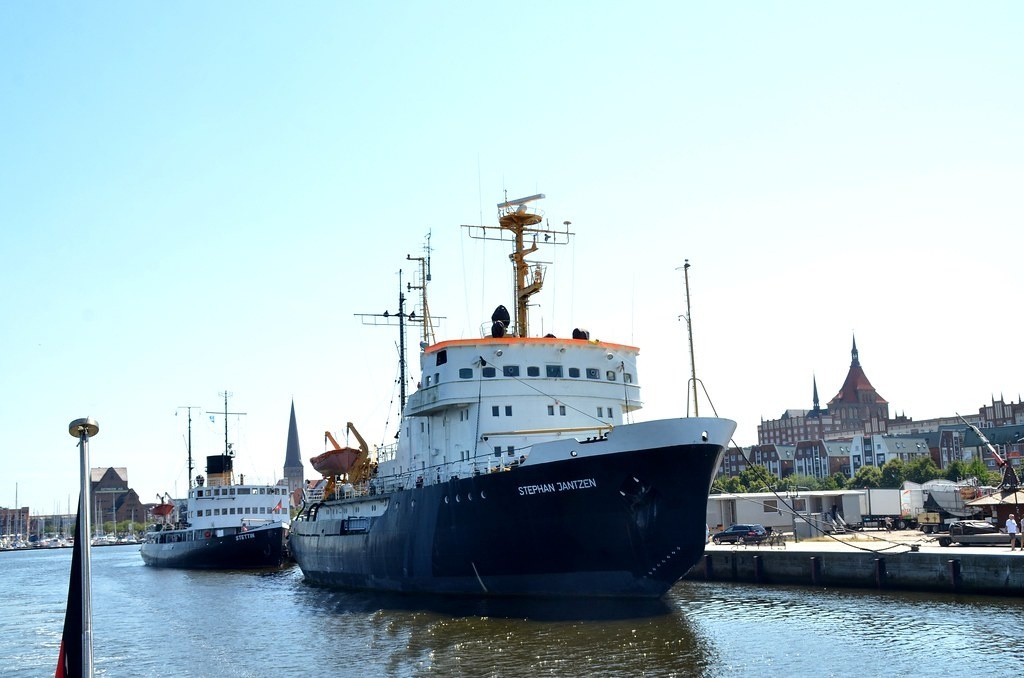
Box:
[1021,548,1023,550]
[1012,549,1016,551]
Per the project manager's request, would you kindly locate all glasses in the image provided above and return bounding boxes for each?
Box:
[1010,516,1013,517]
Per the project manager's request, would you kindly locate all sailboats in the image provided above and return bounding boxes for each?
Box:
[1,492,147,551]
[139,389,295,570]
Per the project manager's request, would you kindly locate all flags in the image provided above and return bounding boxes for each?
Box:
[272,498,281,511]
[54,490,91,678]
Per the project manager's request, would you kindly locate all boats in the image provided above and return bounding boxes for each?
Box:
[288,188,737,600]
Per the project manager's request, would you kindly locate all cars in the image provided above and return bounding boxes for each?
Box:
[712,524,768,545]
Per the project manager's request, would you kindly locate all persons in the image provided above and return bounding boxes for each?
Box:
[449,476,460,482]
[415,476,423,488]
[241,523,247,533]
[1005,514,1018,551]
[1021,514,1024,551]
[830,502,838,520]
